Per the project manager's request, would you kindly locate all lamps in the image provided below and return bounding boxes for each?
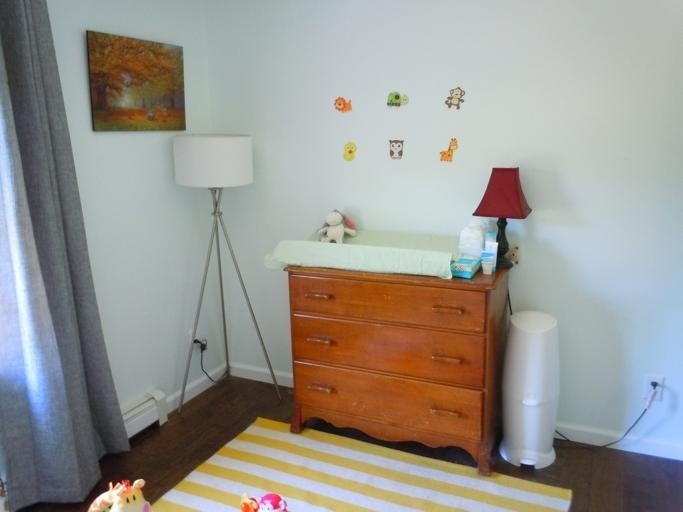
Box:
[471,167,533,269]
[170,135,282,415]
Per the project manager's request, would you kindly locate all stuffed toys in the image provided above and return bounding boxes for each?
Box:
[315,211,357,244]
[86,478,152,512]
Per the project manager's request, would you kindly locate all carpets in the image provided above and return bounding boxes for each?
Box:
[149,416,573,511]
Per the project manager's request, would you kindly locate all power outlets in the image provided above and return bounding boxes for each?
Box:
[640,373,664,401]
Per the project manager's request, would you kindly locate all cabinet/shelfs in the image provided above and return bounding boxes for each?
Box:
[281,265,511,476]
